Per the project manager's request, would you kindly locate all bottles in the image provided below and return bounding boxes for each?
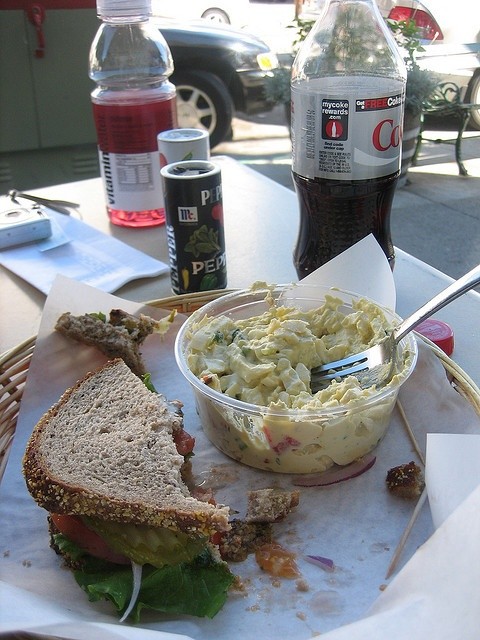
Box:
[88,0,180,229]
[158,129,211,169]
[291,0,408,281]
[161,160,228,295]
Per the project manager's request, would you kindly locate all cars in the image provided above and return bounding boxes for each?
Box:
[136,16,282,147]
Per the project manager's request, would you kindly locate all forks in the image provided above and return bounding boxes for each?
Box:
[307,261,480,394]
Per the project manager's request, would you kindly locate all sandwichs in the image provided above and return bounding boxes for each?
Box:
[22,311,425,623]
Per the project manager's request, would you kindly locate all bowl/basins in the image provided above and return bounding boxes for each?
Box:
[170,283,418,474]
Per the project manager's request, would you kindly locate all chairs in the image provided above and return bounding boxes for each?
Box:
[410,65,479,177]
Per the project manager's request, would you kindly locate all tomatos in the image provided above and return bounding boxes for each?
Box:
[171,426,196,458]
[52,512,128,565]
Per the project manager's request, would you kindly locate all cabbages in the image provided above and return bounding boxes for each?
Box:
[52,508,236,625]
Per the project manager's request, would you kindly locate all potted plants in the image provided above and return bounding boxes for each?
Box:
[285,12,431,191]
